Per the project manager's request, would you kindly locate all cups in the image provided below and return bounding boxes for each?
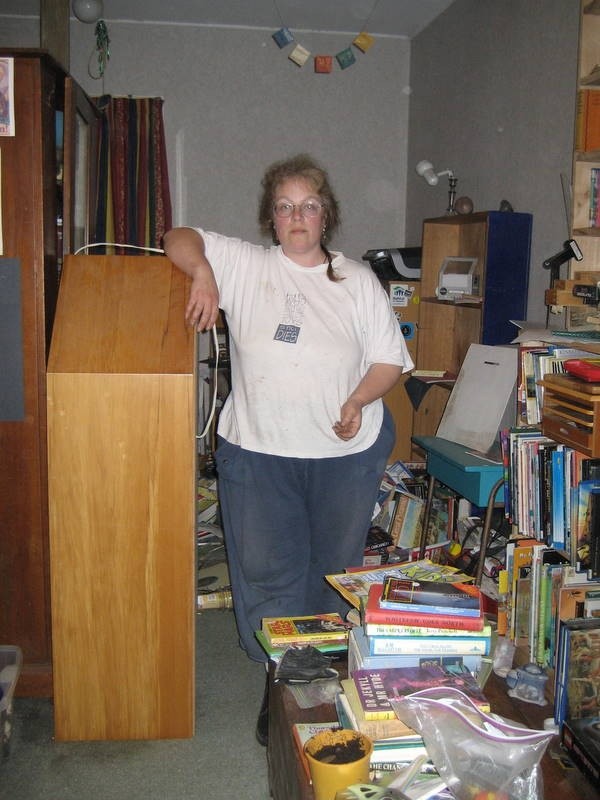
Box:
[304,726,373,800]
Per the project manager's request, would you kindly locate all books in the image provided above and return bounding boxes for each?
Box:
[193,427,600,773]
[572,83,600,232]
[506,265,600,423]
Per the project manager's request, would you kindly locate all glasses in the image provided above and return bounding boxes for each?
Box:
[271,197,326,218]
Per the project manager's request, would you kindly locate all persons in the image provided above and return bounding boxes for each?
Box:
[162,153,414,659]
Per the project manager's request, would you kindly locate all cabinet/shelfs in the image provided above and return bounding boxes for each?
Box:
[533,4,600,465]
[409,210,535,466]
[0,44,109,707]
[378,280,420,469]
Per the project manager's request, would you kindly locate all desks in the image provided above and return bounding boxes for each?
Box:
[268,659,598,799]
